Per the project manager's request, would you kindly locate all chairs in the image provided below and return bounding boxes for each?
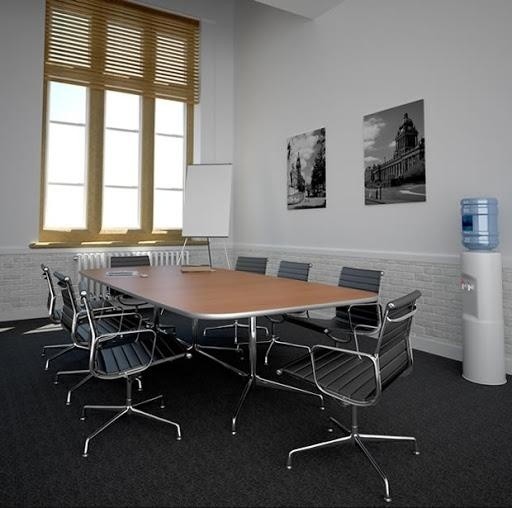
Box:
[55,271,147,407]
[237,260,314,366]
[203,257,272,345]
[42,263,119,371]
[287,289,423,502]
[276,265,385,378]
[78,281,193,457]
[109,255,156,322]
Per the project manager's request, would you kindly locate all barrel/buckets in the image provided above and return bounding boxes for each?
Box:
[460,198,499,250]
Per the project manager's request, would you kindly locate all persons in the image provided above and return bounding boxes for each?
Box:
[397,112,415,136]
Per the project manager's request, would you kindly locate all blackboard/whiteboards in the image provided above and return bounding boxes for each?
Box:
[182,163,232,238]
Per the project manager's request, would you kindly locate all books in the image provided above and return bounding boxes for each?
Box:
[181,267,216,273]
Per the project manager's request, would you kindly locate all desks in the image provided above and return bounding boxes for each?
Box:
[78,266,379,435]
